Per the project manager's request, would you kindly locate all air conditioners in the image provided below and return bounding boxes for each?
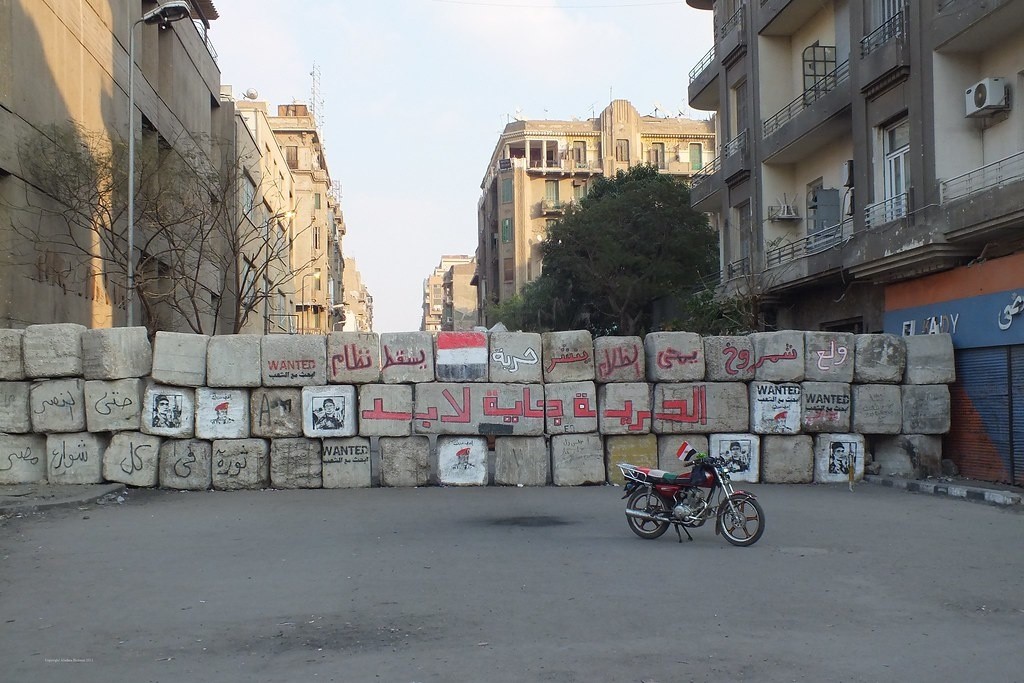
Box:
[964,75,1010,119]
[841,161,854,214]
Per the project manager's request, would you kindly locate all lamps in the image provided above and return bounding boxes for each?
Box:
[499,159,511,171]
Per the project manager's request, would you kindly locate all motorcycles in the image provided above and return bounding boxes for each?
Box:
[616,452,765,547]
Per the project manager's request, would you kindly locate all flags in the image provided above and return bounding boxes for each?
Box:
[676,441,698,461]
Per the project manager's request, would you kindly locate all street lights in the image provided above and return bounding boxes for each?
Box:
[263,210,298,335]
[125,1,191,327]
[302,271,321,334]
